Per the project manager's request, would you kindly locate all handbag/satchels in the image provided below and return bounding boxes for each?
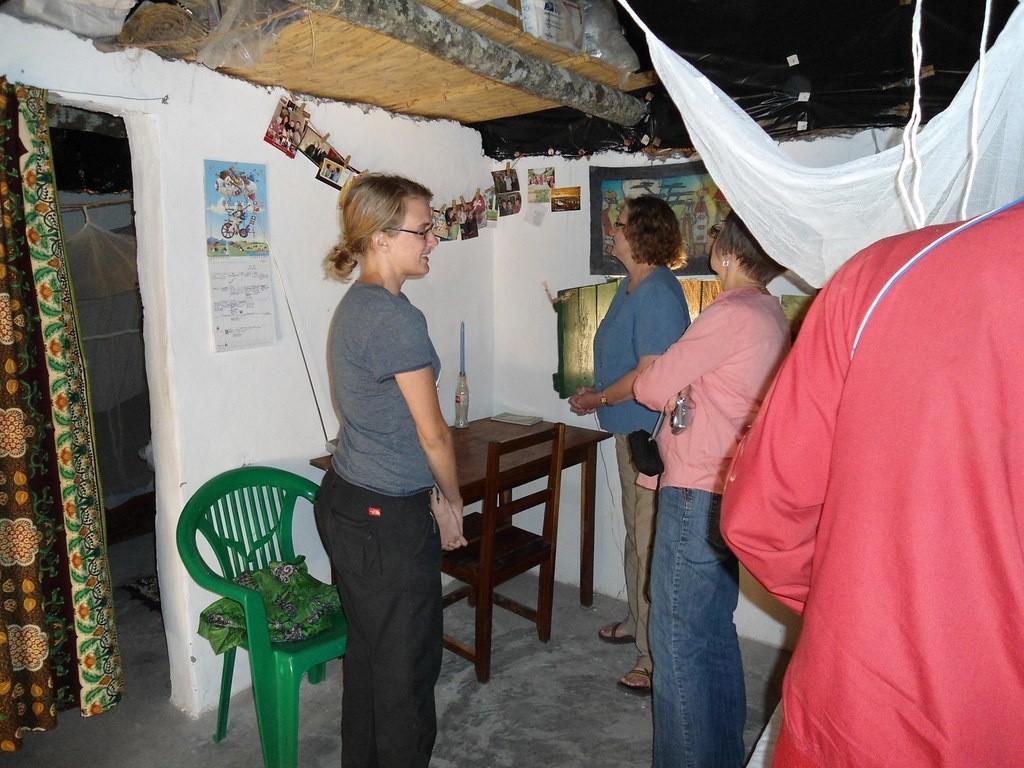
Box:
[629,429,664,476]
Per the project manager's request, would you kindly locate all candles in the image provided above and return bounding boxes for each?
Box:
[460,321,466,373]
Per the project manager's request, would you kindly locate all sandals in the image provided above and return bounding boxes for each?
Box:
[616,665,653,696]
[598,621,636,644]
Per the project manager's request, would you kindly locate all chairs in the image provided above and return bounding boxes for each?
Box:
[177,421,566,768]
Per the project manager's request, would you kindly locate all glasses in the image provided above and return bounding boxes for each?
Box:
[711,224,723,238]
[382,223,434,239]
[613,222,626,229]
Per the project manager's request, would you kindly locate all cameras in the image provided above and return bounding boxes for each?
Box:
[670,397,687,435]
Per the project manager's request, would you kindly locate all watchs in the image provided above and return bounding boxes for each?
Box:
[601,389,610,406]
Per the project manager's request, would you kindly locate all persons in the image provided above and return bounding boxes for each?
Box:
[444,170,521,239]
[719,196,1024,768]
[632,209,788,768]
[272,106,300,152]
[310,173,468,768]
[306,140,320,161]
[324,164,340,182]
[568,194,691,696]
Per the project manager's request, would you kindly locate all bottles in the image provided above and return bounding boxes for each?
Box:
[454,376,469,428]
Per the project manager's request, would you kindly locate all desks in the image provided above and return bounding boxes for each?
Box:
[311,410,615,609]
